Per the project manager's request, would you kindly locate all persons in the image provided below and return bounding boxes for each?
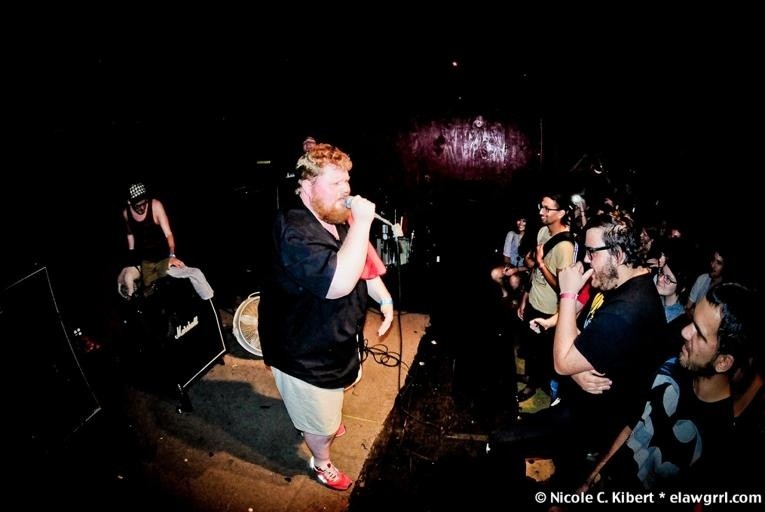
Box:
[491,187,764,512]
[303,135,316,153]
[116,183,183,294]
[257,142,394,490]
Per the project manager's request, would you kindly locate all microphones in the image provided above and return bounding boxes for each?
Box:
[344,193,395,232]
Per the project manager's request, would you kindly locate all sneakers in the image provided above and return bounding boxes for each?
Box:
[301,424,346,437]
[310,456,353,491]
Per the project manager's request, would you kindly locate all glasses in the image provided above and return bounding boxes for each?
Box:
[585,246,611,254]
[658,270,677,286]
[538,204,559,214]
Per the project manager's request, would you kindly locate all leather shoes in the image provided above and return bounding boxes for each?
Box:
[517,374,529,384]
[517,388,536,402]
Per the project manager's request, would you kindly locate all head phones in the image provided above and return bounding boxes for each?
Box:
[125,199,132,206]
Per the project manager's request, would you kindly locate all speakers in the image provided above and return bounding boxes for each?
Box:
[126,272,230,416]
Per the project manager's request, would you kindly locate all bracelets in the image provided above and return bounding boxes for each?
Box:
[169,253,175,258]
[378,299,393,305]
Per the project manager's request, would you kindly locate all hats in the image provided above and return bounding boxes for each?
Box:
[129,184,146,204]
